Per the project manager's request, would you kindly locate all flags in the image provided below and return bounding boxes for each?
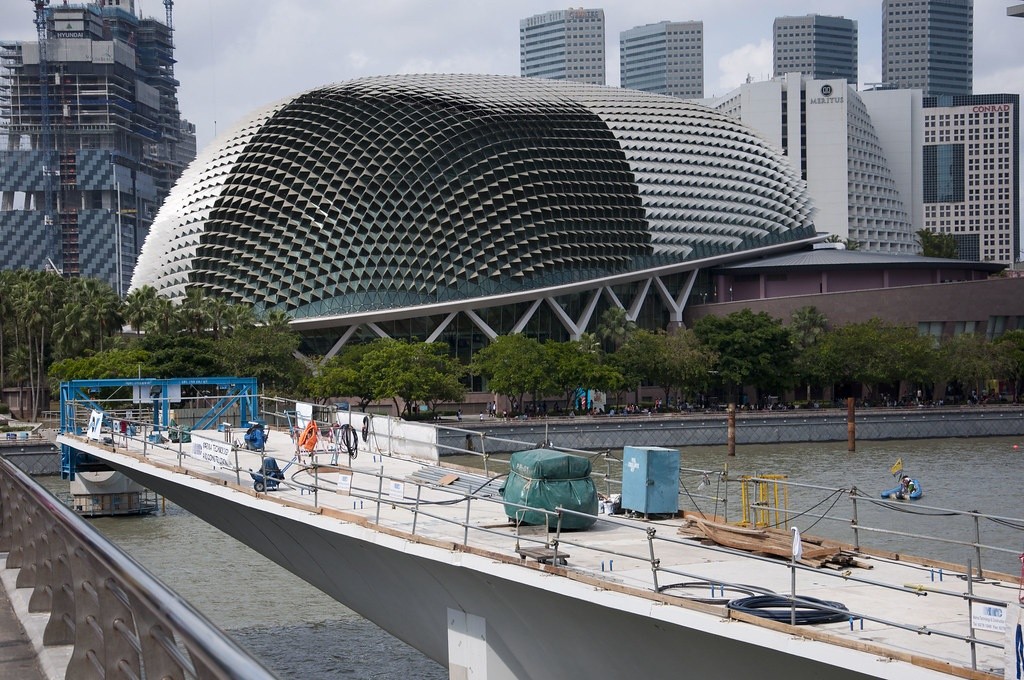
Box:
[892,459,904,480]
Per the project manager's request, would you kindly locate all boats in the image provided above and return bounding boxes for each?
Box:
[881,479,922,500]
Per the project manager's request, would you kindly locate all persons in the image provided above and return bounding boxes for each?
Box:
[501,386,1024,420]
[902,474,917,496]
[415,404,418,415]
[417,404,421,415]
[480,411,486,422]
[488,401,494,418]
[493,400,498,418]
[458,410,464,422]
[334,421,340,437]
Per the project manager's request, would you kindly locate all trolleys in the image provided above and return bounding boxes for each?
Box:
[246,425,272,452]
[250,457,297,492]
[513,506,571,567]
